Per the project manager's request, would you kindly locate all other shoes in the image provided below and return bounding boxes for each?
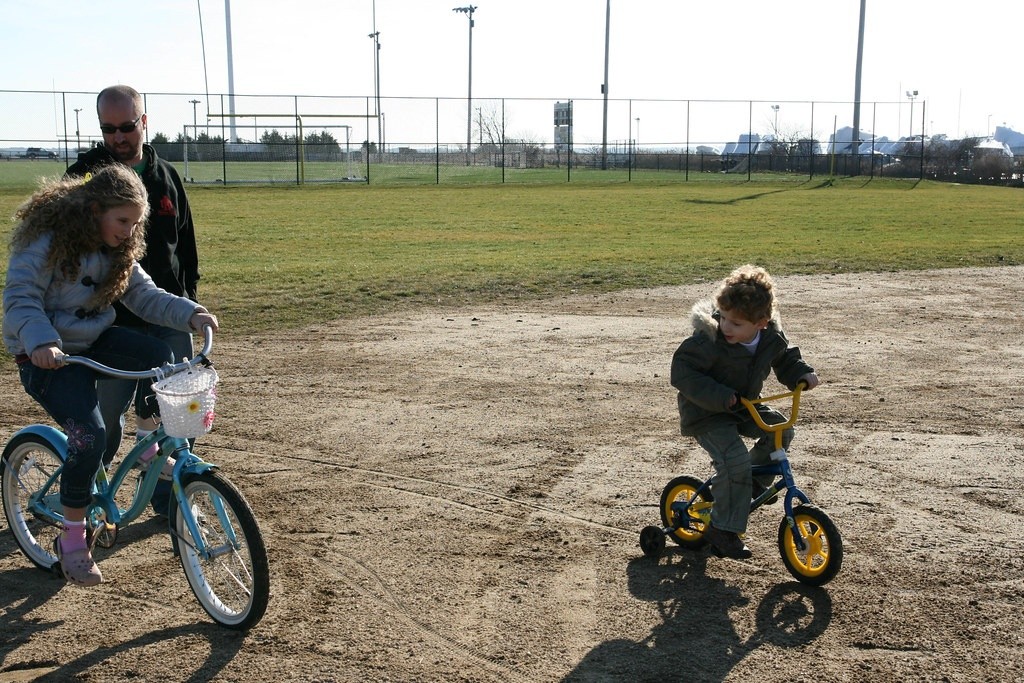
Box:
[159,504,206,526]
[702,521,752,560]
[752,481,778,505]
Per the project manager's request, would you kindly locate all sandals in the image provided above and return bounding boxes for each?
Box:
[53,533,103,586]
[132,452,176,480]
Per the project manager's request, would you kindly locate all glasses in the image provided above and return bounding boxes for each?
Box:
[100,118,140,134]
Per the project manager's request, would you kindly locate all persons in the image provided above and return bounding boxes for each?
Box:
[670,264,820,559]
[61,84,199,517]
[2,162,219,588]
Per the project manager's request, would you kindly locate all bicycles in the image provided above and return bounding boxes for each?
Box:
[641,379,843,586]
[0,323,270,631]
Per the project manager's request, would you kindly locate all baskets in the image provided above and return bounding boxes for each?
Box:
[151,357,219,438]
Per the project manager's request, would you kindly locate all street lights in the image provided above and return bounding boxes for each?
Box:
[207,118,211,140]
[906,90,918,141]
[189,99,201,152]
[368,31,382,164]
[635,118,640,153]
[452,5,478,165]
[772,105,780,135]
[74,108,80,152]
[381,113,385,153]
[988,114,992,139]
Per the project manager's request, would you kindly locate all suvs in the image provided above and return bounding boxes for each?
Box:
[26,147,57,159]
